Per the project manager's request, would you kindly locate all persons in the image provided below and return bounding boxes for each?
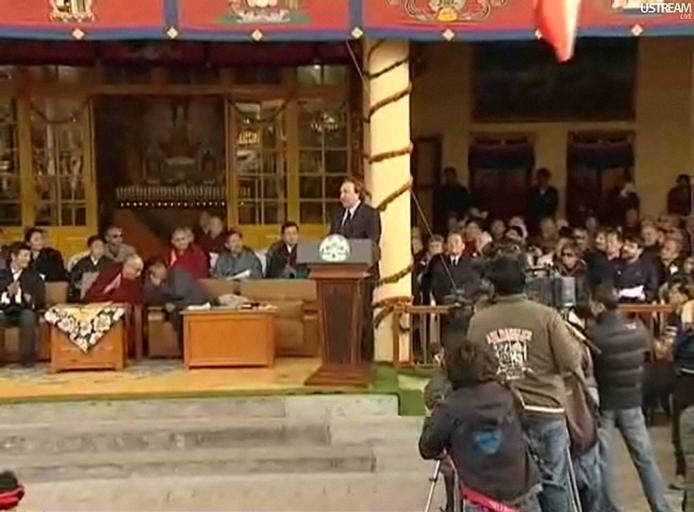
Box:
[184,227,210,277]
[264,221,312,278]
[144,256,219,358]
[564,348,621,511]
[215,229,263,279]
[104,226,136,263]
[525,254,587,312]
[329,177,382,362]
[81,254,140,304]
[466,254,589,512]
[583,281,667,512]
[417,341,555,512]
[591,170,694,279]
[197,216,228,256]
[164,226,209,277]
[410,166,467,305]
[193,211,211,240]
[654,278,693,490]
[68,236,116,302]
[469,167,602,254]
[0,240,48,367]
[26,228,67,277]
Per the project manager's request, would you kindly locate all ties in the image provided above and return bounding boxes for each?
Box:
[344,209,352,228]
[453,260,456,264]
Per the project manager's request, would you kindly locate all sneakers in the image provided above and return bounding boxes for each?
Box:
[669,474,685,492]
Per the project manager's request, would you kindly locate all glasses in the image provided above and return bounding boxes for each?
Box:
[113,235,123,238]
[562,254,574,257]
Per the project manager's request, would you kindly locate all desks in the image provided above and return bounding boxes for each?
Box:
[45,300,130,374]
[180,301,275,370]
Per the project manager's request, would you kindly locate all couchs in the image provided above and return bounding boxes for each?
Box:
[146,278,322,358]
[1,280,69,364]
